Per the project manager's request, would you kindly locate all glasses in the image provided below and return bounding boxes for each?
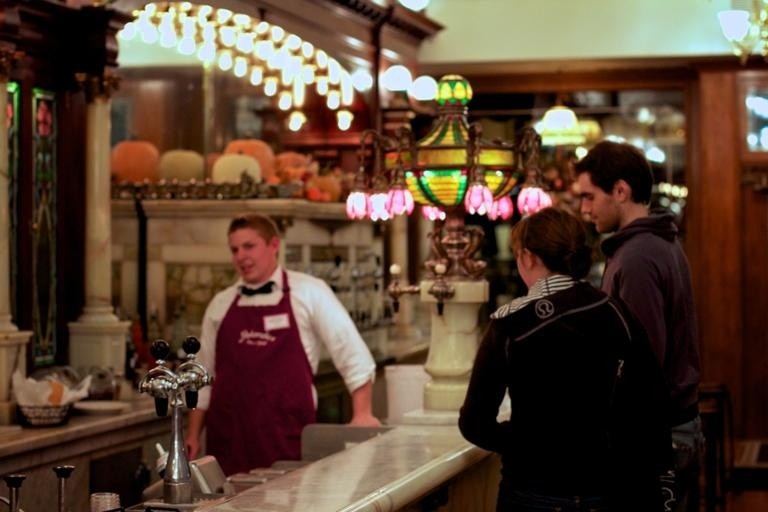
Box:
[716,1,768,67]
[344,71,553,224]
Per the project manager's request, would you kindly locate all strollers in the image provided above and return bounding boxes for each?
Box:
[238,280,275,298]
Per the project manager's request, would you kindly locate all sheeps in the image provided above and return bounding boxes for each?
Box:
[138,337,216,417]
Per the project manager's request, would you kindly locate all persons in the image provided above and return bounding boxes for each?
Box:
[566,138,708,512]
[456,205,683,512]
[180,212,383,481]
